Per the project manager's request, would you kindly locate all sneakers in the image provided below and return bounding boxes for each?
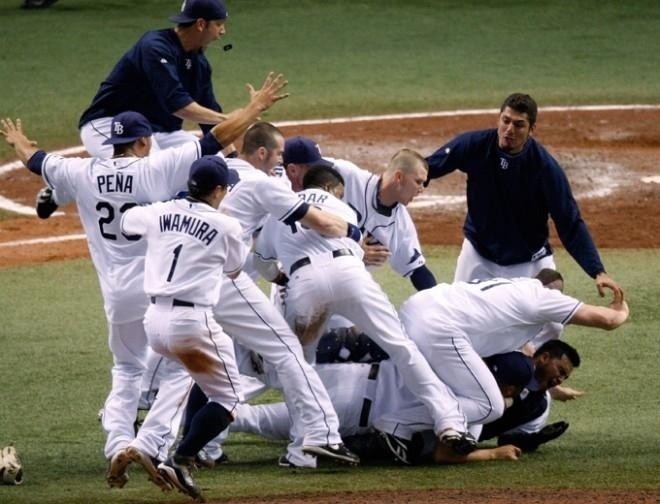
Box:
[193,454,230,466]
[441,431,499,451]
[250,349,265,375]
[2,445,25,485]
[157,457,201,499]
[97,408,144,438]
[35,186,58,219]
[125,446,174,492]
[278,455,296,469]
[301,443,361,467]
[106,449,133,489]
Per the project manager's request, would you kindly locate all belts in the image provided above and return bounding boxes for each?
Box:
[359,364,379,427]
[289,248,352,276]
[150,296,194,307]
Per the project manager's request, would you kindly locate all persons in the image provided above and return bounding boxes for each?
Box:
[79,0,262,161]
[418,90,625,358]
[0,71,290,489]
[126,123,633,501]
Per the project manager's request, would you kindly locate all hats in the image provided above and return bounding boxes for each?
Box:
[168,1,228,24]
[189,155,239,189]
[282,136,334,169]
[102,111,154,145]
